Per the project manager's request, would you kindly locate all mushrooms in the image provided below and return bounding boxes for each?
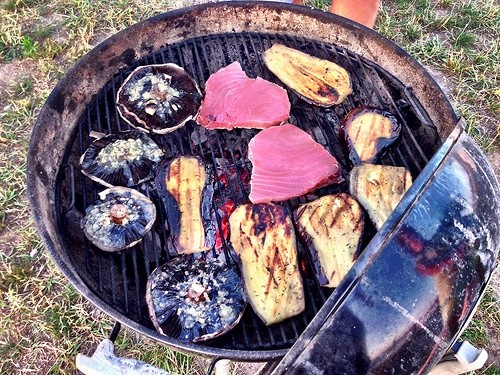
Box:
[78,129,164,189]
[79,185,159,251]
[144,254,246,345]
[116,63,205,135]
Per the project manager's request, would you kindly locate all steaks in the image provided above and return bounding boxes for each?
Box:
[197,60,294,129]
[247,123,345,205]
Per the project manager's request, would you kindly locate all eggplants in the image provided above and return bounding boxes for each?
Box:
[292,190,366,288]
[349,160,417,230]
[261,43,353,107]
[227,203,307,325]
[341,107,403,166]
[154,155,216,255]
